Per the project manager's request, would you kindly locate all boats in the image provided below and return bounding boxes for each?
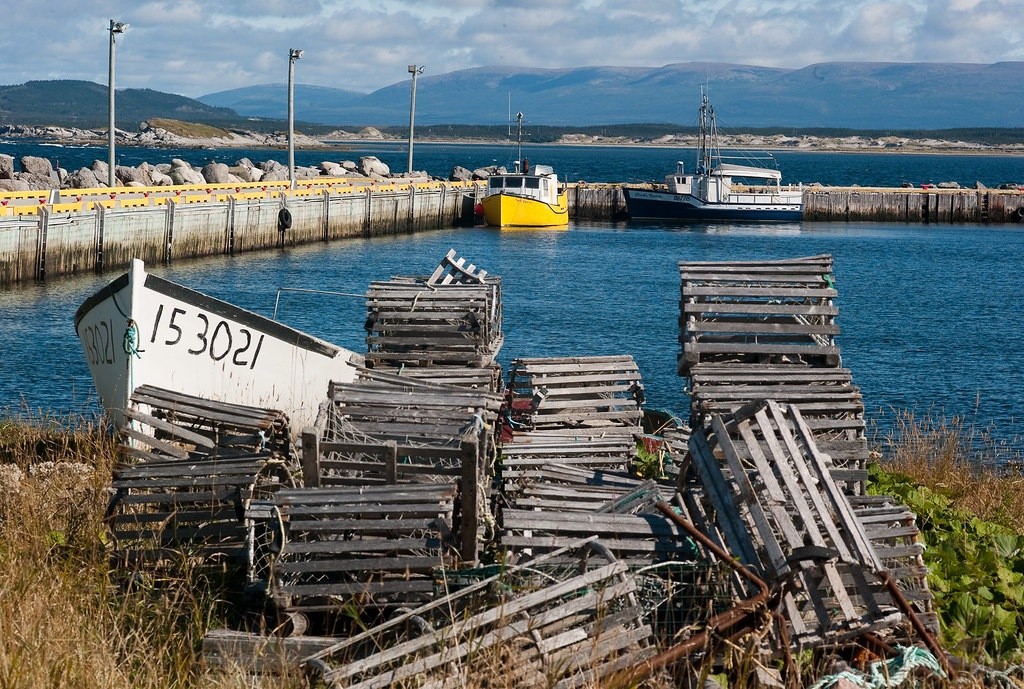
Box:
[72,258,369,444]
[481,112,570,228]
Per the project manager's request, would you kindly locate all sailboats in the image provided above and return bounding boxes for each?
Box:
[622,85,807,225]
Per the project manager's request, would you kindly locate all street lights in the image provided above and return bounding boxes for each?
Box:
[286,47,304,182]
[407,65,427,173]
[108,19,130,189]
